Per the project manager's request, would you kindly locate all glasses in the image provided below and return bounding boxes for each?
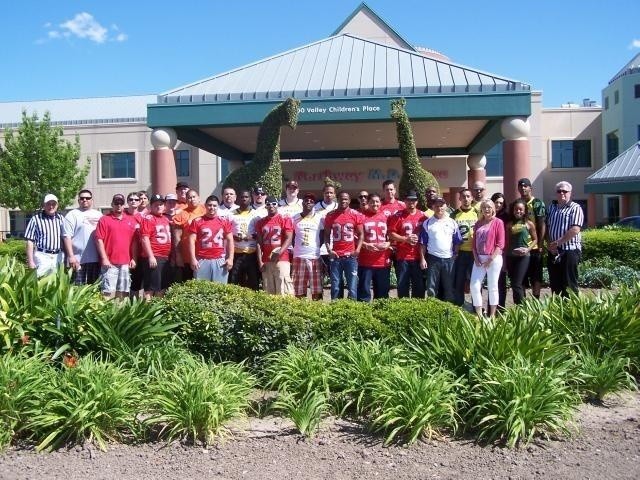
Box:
[305,199,314,204]
[257,193,265,195]
[358,195,368,199]
[266,203,277,206]
[556,189,570,193]
[128,198,141,202]
[114,202,125,205]
[79,196,92,200]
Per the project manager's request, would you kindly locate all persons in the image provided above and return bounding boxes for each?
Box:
[25,194,65,277]
[470,199,505,318]
[65,180,545,302]
[543,180,584,300]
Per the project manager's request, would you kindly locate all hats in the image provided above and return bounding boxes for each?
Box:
[44,194,58,205]
[266,196,282,207]
[285,181,298,188]
[112,194,124,201]
[519,178,531,187]
[175,183,188,189]
[303,192,315,201]
[434,198,445,204]
[165,194,179,202]
[150,195,167,203]
[404,191,418,200]
[254,187,266,194]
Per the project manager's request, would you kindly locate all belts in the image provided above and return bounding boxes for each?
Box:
[38,248,60,254]
